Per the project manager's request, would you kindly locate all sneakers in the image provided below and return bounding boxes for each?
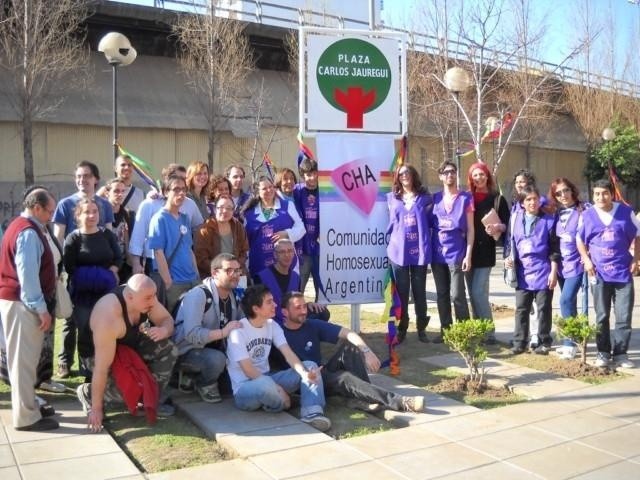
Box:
[432,335,444,343]
[300,413,331,432]
[614,354,635,368]
[346,399,380,411]
[15,394,58,431]
[596,352,610,367]
[556,345,581,360]
[535,344,551,354]
[76,384,92,416]
[57,363,70,379]
[178,370,222,403]
[132,403,175,416]
[399,396,425,412]
[40,380,66,392]
[509,347,524,355]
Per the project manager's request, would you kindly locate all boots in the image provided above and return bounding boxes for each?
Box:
[395,317,409,344]
[418,316,429,343]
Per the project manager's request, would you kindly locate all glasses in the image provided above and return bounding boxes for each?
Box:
[441,170,456,175]
[214,268,243,277]
[553,187,571,198]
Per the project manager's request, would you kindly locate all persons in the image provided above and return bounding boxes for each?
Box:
[385,160,510,345]
[0,155,330,433]
[503,168,640,367]
[271,291,424,413]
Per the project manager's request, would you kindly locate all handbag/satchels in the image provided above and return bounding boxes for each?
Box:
[504,256,518,288]
[149,270,166,309]
[55,271,75,319]
[550,208,560,253]
[494,193,504,246]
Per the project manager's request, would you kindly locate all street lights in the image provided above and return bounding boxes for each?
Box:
[485,116,500,176]
[97,31,139,177]
[604,128,617,173]
[442,66,471,193]
